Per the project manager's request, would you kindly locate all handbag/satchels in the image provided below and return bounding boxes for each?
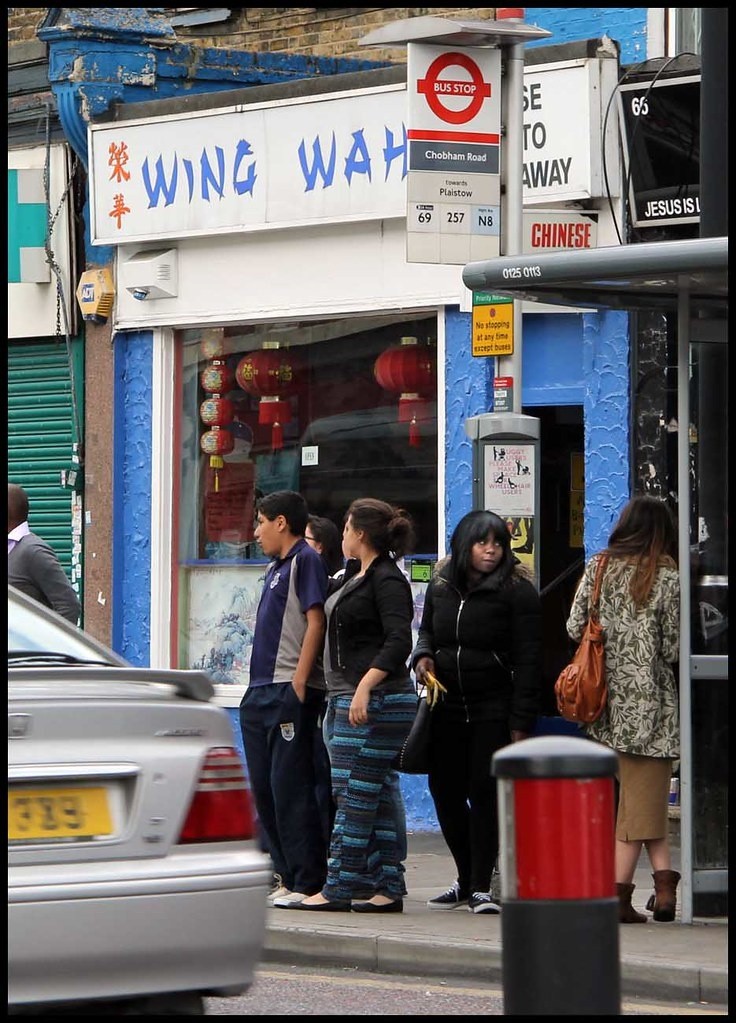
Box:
[390,675,439,774]
[553,552,609,724]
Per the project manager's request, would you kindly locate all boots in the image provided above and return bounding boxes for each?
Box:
[650,869,681,922]
[615,882,648,924]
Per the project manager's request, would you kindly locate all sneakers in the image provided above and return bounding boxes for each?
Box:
[426,880,470,909]
[466,890,502,914]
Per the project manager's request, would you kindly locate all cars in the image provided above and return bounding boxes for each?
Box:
[8,581,273,1016]
[296,395,438,549]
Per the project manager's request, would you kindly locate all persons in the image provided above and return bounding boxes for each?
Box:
[287,499,419,912]
[8,483,80,625]
[333,525,410,582]
[412,511,543,915]
[239,492,332,908]
[305,516,343,578]
[566,495,681,924]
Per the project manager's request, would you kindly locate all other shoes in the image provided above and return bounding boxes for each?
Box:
[352,898,403,913]
[273,892,311,909]
[288,898,352,912]
[266,873,290,907]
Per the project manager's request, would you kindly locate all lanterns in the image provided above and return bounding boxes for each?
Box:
[199,398,235,430]
[202,365,234,399]
[201,328,234,365]
[200,430,235,493]
[235,340,299,450]
[374,336,437,446]
